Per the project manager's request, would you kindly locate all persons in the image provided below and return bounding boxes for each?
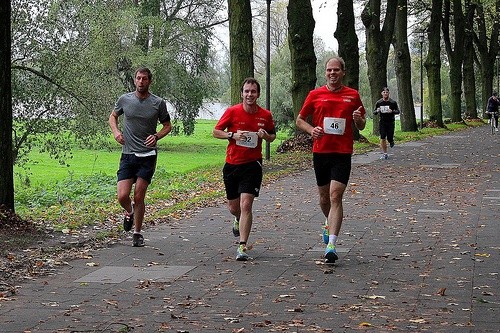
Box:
[111,69,172,247]
[373,87,400,160]
[296,56,366,263]
[213,79,276,260]
[486,93,500,132]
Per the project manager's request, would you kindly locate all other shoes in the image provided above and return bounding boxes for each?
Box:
[488,120,491,124]
[495,128,499,132]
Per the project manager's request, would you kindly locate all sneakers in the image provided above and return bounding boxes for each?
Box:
[131,232,145,247]
[389,143,394,148]
[124,201,135,231]
[379,154,388,160]
[323,243,339,263]
[233,217,240,237]
[321,219,329,244]
[235,244,248,261]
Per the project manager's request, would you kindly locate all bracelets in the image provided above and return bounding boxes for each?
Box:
[153,134,158,141]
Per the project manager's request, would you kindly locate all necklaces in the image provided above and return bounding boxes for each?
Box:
[326,84,342,91]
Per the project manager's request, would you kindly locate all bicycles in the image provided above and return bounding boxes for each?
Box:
[485,112,500,134]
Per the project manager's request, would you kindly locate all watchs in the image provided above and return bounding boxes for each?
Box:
[228,131,234,141]
